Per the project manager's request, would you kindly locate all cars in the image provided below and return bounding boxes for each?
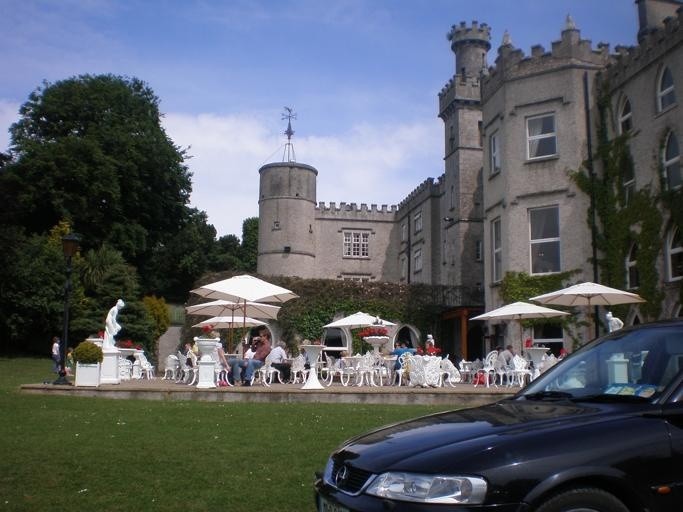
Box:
[311,318,682,512]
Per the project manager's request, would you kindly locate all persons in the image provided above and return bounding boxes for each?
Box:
[103,299,125,350]
[495,344,515,386]
[297,329,439,387]
[52,336,75,376]
[605,311,627,361]
[437,358,440,359]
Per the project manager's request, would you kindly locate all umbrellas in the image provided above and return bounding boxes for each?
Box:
[185,274,300,385]
[529,281,647,386]
[322,311,397,356]
[469,302,572,356]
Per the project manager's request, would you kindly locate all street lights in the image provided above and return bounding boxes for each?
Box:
[51,228,81,387]
